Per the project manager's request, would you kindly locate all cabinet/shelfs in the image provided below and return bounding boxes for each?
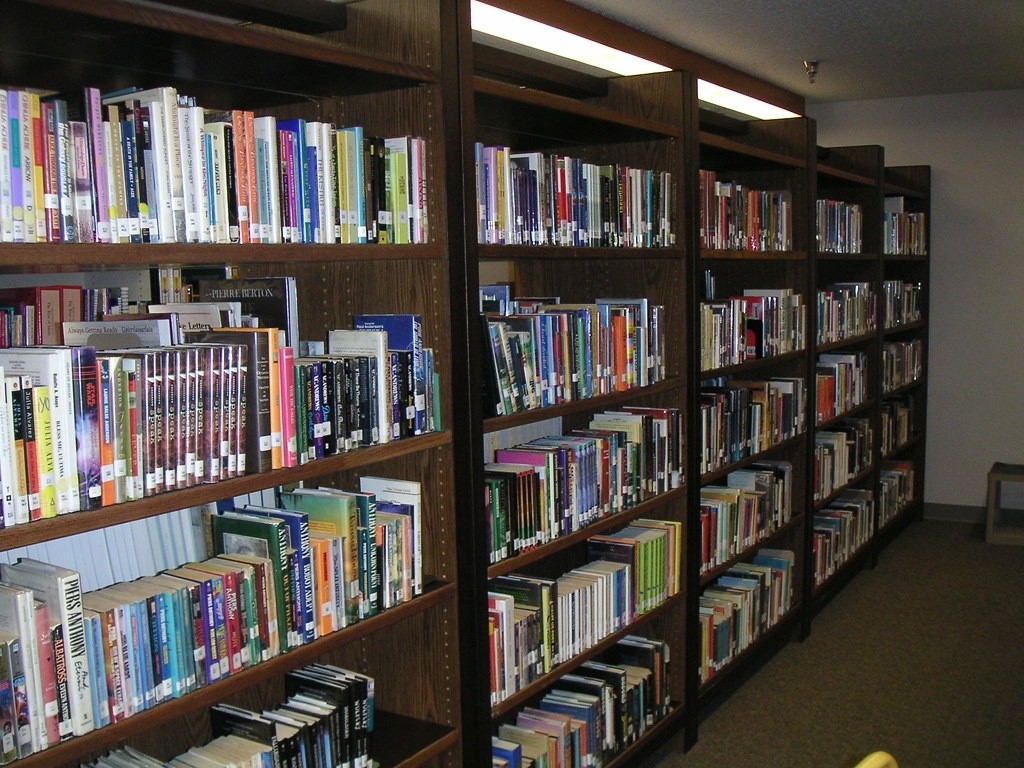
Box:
[0,0,931,768]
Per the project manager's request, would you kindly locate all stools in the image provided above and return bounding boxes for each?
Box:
[986,461,1024,546]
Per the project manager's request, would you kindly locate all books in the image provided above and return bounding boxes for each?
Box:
[813,489,874,592]
[0,263,441,530]
[816,339,922,427]
[484,406,685,565]
[0,86,429,243]
[698,460,795,688]
[701,373,807,476]
[816,199,927,257]
[878,460,914,530]
[488,519,682,768]
[817,280,923,344]
[475,141,677,248]
[880,393,920,458]
[699,169,793,251]
[0,476,421,768]
[698,268,807,372]
[814,416,874,507]
[478,281,665,416]
